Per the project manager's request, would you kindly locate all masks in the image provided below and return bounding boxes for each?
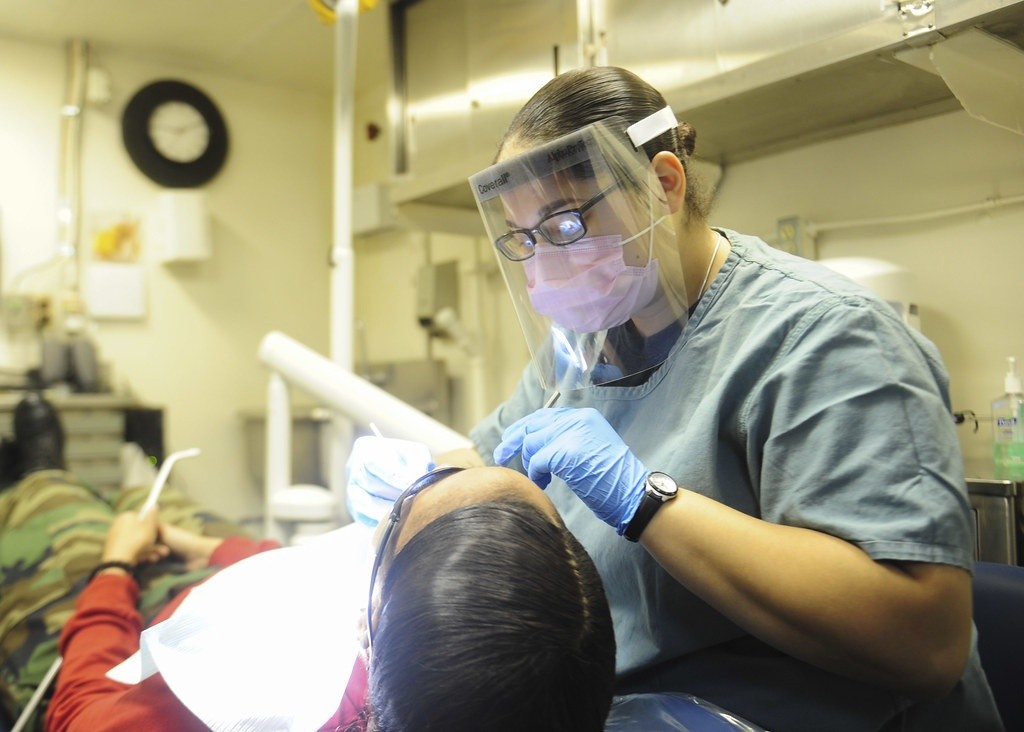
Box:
[521,168,672,333]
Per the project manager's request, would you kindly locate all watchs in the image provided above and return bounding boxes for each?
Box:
[623,470,678,543]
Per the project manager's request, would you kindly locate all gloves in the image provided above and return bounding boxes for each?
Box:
[494,407,648,536]
[346,435,436,532]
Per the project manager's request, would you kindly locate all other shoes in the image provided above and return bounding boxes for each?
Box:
[13,395,64,475]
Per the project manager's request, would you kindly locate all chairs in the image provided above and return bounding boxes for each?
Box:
[973,560,1024,732]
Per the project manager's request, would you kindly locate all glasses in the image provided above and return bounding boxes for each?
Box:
[365,465,467,663]
[493,158,655,263]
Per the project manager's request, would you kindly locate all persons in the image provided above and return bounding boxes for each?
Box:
[345,65,1004,732]
[0,389,617,731]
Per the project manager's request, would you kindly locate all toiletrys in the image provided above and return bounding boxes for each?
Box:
[990,353,1022,483]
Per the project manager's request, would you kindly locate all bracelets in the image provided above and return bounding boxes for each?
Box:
[88,561,146,589]
